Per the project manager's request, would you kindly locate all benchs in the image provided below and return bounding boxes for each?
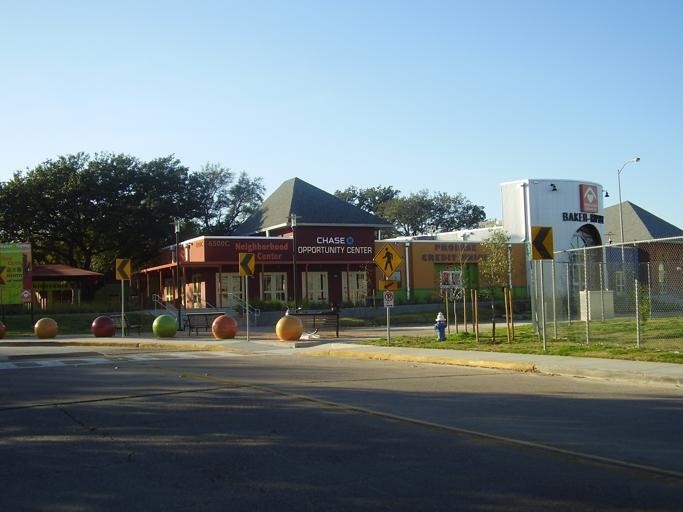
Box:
[110,314,142,337]
[186,312,226,336]
[287,312,340,338]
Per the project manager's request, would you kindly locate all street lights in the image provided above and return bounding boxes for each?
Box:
[616,155,641,314]
[285,211,303,313]
[170,216,185,331]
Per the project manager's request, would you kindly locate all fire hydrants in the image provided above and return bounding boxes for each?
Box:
[432,311,448,342]
